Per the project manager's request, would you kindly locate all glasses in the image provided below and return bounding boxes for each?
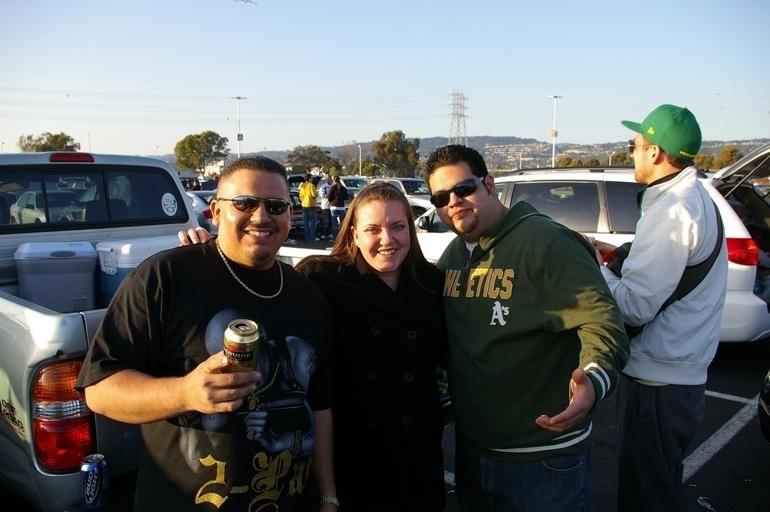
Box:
[628,139,654,154]
[217,195,290,216]
[430,176,485,208]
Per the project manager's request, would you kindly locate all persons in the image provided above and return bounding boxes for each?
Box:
[297,171,351,244]
[184,181,194,192]
[191,180,202,192]
[73,154,342,511]
[177,180,449,507]
[581,103,732,510]
[422,142,632,510]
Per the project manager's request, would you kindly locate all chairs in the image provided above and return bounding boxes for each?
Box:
[83,198,128,224]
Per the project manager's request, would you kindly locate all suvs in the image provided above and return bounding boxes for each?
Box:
[59,176,130,224]
[414,140,770,346]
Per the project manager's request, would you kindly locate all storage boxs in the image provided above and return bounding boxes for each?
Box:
[13,240,98,314]
[95,235,181,307]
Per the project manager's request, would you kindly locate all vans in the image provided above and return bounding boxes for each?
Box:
[288,172,322,189]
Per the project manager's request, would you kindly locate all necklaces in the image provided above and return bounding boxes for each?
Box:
[215,235,285,300]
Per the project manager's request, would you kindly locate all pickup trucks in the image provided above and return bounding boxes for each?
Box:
[0,153,332,512]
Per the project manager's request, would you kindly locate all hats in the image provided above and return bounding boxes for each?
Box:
[621,104,702,162]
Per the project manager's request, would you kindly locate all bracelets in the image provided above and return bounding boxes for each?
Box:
[320,494,342,508]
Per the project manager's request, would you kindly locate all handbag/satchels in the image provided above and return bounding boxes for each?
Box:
[605,200,723,339]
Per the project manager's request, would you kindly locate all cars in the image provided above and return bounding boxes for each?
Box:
[757,370,770,448]
[755,184,770,201]
[178,175,219,238]
[0,192,17,222]
[369,178,434,219]
[56,174,92,190]
[287,189,322,231]
[333,177,368,206]
[8,190,84,225]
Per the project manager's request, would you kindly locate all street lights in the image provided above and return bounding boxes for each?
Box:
[356,143,363,175]
[548,92,565,168]
[230,95,248,159]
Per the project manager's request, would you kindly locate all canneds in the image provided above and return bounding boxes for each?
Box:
[221,319,258,375]
[80,454,109,510]
